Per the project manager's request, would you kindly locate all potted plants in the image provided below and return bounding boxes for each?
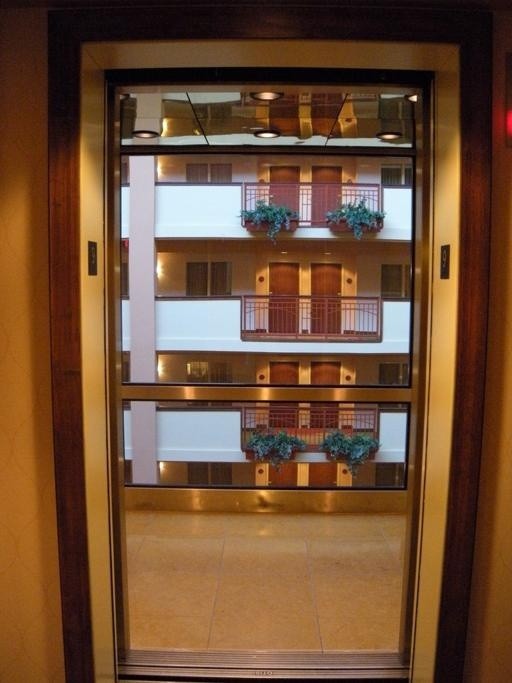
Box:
[246,429,307,467]
[326,198,386,240]
[319,430,382,475]
[239,200,299,242]
